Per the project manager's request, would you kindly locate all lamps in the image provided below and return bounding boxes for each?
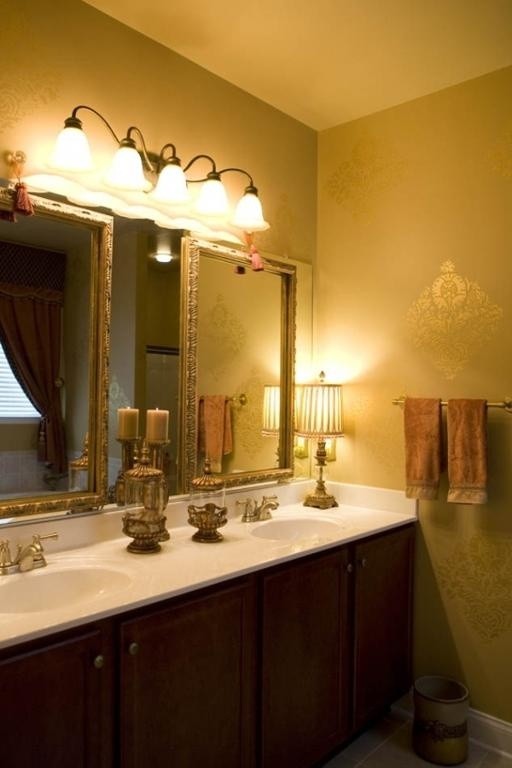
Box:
[260,386,280,468]
[53,105,270,236]
[294,371,348,511]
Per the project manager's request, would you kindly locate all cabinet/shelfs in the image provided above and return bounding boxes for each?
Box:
[0,567,257,766]
[259,520,417,766]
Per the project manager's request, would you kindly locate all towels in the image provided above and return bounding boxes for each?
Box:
[445,399,489,506]
[203,394,233,474]
[403,394,445,501]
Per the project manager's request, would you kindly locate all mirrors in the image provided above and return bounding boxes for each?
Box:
[177,236,298,487]
[0,189,116,518]
[109,213,180,504]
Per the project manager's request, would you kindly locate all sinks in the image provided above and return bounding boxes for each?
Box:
[0,567,132,616]
[249,517,338,543]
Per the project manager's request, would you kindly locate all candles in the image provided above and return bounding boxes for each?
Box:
[118,405,140,439]
[144,406,168,443]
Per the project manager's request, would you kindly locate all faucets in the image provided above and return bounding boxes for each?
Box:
[255,502,280,516]
[13,543,44,574]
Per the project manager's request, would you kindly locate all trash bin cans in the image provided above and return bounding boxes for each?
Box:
[411,676,469,766]
